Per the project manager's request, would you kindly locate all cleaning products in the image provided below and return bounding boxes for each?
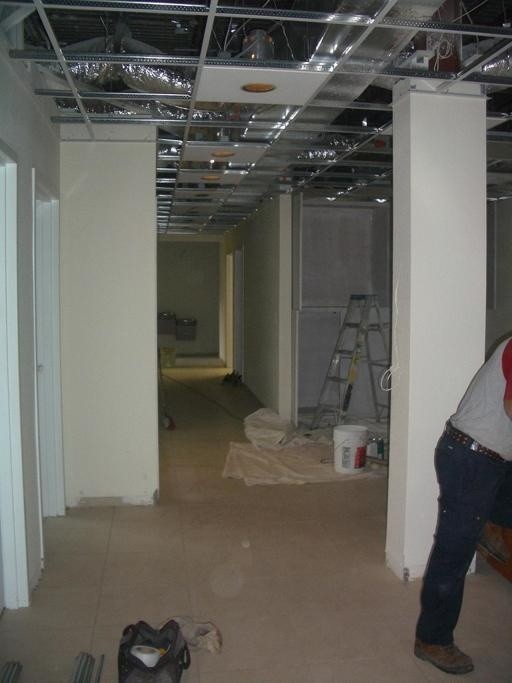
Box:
[370,436,385,461]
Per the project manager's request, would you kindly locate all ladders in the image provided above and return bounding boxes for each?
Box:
[310,294,390,429]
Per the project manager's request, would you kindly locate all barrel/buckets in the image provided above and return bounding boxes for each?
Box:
[176,318,196,341]
[330,424,369,475]
[157,311,176,334]
[242,28,275,61]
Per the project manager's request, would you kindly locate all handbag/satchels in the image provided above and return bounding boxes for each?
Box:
[118,620,190,683]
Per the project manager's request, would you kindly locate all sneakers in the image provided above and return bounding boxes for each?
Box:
[415,637,474,674]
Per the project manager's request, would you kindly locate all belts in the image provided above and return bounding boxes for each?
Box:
[445,421,499,460]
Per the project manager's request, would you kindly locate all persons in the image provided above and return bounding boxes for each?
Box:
[411,331,512,675]
